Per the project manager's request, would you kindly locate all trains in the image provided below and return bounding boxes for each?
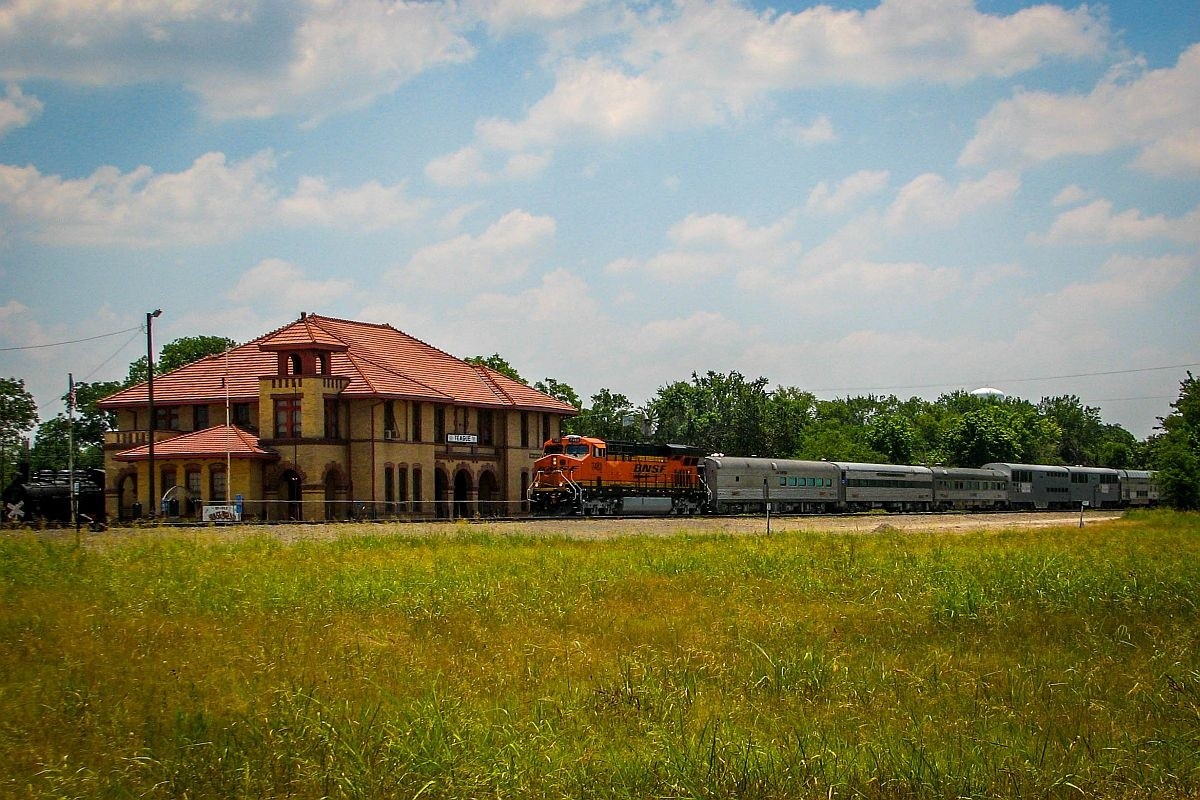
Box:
[526,435,1163,517]
[5,459,107,525]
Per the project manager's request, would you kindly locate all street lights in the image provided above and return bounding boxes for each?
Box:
[146,307,162,520]
